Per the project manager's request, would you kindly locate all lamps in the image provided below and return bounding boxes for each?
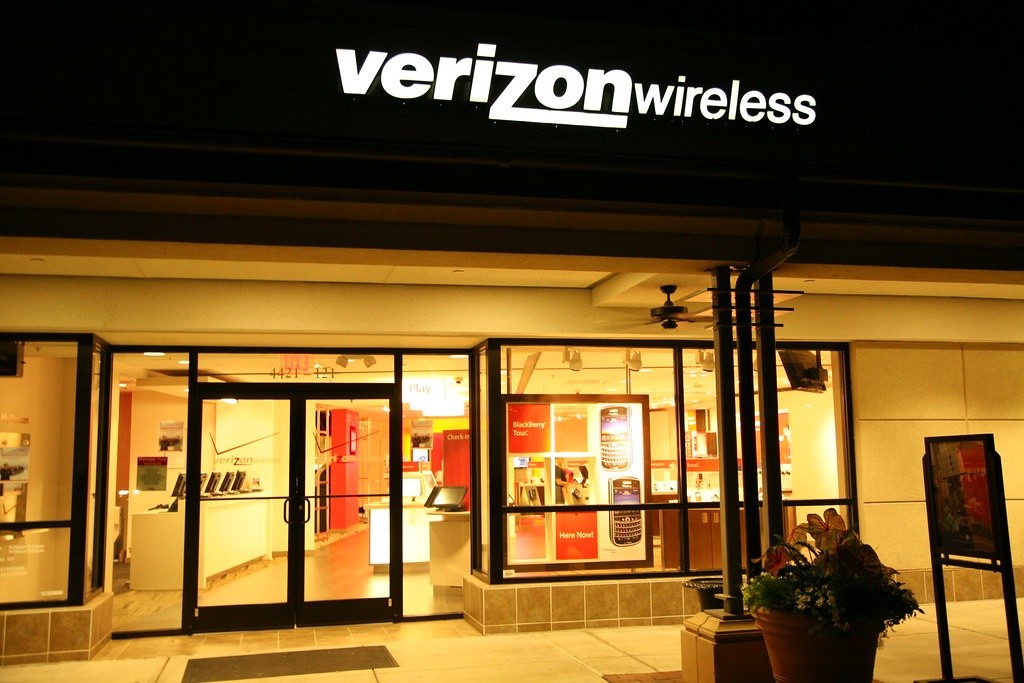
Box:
[569,347,583,372]
[627,349,642,372]
[753,357,758,373]
[702,351,716,372]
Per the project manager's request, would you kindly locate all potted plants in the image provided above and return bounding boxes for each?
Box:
[742,506,925,683]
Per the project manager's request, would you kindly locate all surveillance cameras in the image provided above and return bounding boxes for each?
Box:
[456,377,463,384]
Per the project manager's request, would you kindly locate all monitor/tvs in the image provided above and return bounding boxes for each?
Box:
[432,486,468,512]
[778,350,828,393]
[514,457,532,469]
[172,470,247,500]
[424,486,443,510]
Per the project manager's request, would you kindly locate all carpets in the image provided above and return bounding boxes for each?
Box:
[182,644,401,683]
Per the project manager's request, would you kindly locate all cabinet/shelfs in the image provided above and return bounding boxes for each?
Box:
[658,509,746,571]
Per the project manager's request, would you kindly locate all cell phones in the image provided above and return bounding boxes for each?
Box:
[608,476,643,547]
[600,406,632,472]
[573,488,582,499]
[529,489,537,500]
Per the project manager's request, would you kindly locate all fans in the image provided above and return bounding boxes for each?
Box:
[591,284,754,330]
